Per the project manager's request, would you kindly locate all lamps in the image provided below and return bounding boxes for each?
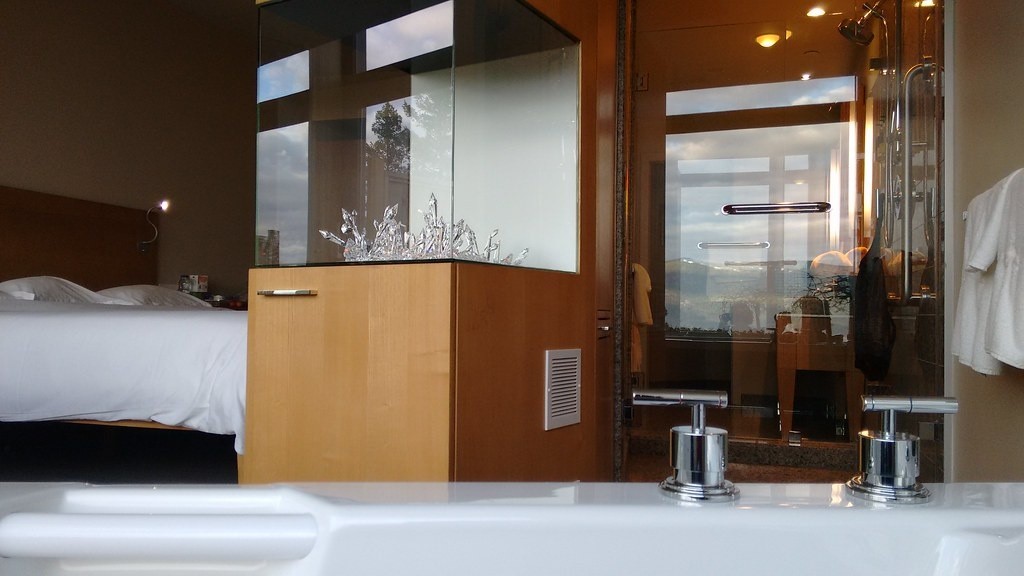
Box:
[138,200,170,253]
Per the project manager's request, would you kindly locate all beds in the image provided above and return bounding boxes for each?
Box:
[0,184,249,455]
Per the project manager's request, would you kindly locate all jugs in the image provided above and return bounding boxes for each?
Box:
[178,275,192,293]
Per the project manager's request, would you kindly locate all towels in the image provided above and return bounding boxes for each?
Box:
[949,166,1024,379]
[629,325,643,373]
[630,262,653,327]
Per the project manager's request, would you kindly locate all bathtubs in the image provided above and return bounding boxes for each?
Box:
[0,481,1024,575]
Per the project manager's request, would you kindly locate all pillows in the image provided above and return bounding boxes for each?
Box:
[93,284,211,306]
[0,276,111,305]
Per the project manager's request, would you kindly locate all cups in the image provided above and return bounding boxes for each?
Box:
[214,295,223,301]
[921,285,931,298]
